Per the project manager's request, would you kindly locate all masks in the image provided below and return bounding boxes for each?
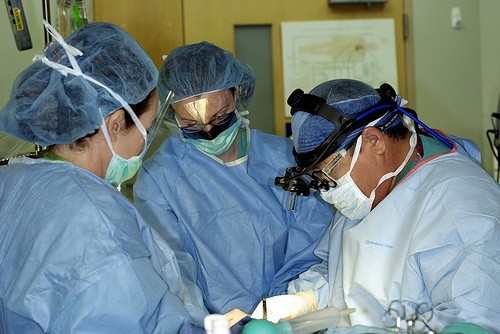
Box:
[177,107,243,156]
[320,136,384,221]
[104,121,148,187]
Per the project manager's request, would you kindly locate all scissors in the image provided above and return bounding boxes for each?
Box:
[380,296,434,334]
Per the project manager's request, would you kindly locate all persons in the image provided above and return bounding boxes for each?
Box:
[133,41,336,316]
[0,22,205,334]
[252,79,500,334]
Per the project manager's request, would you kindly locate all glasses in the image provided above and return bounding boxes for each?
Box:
[300,125,383,188]
[173,101,236,134]
